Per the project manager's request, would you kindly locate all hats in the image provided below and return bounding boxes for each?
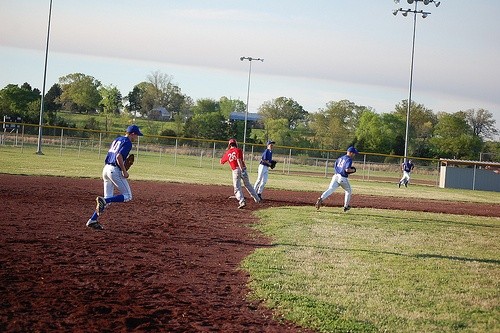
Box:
[229,139,236,145]
[347,147,357,153]
[266,140,275,145]
[127,125,144,136]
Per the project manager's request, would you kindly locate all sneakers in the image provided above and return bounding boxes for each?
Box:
[85,218,106,231]
[96,196,107,216]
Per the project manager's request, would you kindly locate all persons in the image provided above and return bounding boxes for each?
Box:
[85,124,144,230]
[315,147,360,212]
[398,159,414,189]
[220,138,260,209]
[251,141,276,200]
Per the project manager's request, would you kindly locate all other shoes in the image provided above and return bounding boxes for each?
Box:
[254,193,262,203]
[343,205,351,211]
[315,197,323,211]
[399,182,401,188]
[404,181,408,187]
[237,201,246,209]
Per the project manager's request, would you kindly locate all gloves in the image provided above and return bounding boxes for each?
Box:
[241,171,248,179]
[271,160,277,169]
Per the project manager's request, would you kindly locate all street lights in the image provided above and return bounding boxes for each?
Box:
[239,57,265,162]
[393,0,441,169]
